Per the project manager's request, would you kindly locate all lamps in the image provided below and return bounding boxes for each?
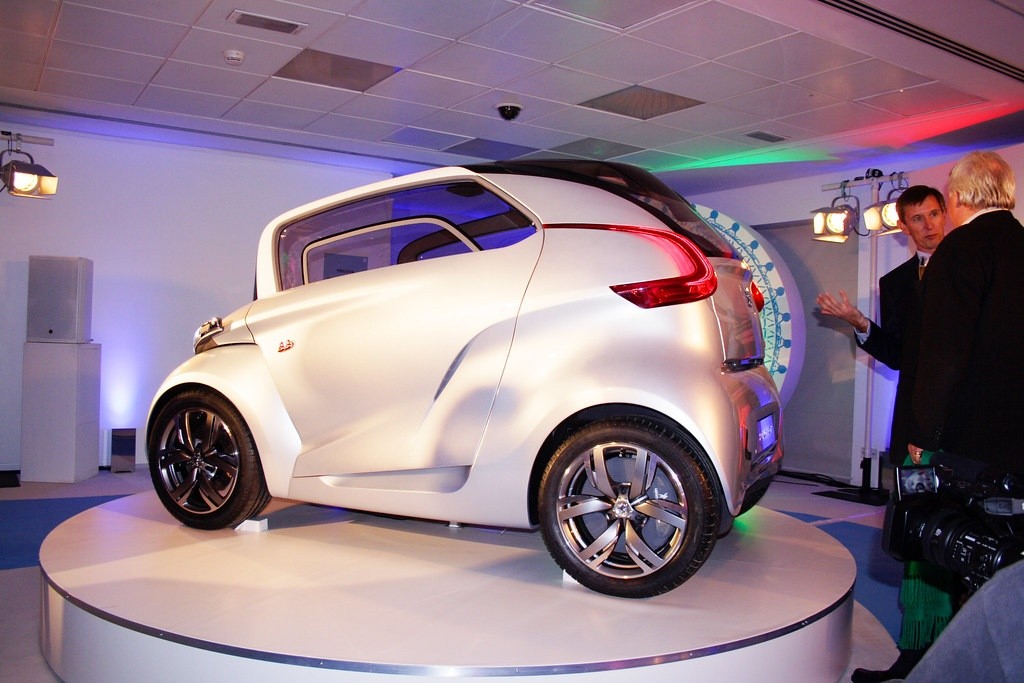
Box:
[810,196,859,243]
[863,187,909,237]
[0,149,59,200]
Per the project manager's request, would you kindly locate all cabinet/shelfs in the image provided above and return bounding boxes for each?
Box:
[20,343,101,483]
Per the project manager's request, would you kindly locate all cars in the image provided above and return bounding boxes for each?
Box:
[144,159,783,599]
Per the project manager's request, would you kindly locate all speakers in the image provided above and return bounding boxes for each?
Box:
[26,255,93,343]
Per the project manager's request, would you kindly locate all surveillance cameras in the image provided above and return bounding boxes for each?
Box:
[495,103,525,120]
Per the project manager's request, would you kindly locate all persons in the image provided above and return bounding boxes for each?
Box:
[907,150,1024,614]
[815,184,954,683]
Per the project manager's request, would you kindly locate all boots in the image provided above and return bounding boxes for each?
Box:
[851,621,936,683]
